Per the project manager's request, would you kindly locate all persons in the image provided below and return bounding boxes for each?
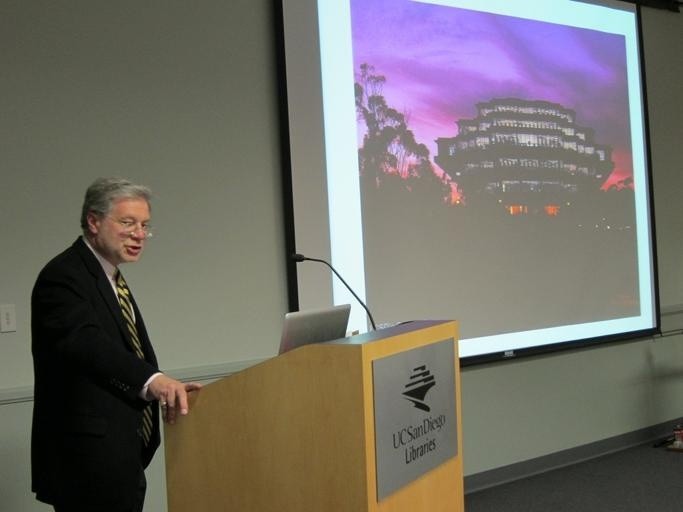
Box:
[31,179,203,511]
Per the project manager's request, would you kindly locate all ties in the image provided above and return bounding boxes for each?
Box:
[117,266,153,446]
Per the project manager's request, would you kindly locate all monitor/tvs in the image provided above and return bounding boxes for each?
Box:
[278,304,351,355]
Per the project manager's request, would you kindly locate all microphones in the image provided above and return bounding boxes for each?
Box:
[293,252,376,331]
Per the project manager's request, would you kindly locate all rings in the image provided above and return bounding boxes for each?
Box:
[161,402,166,406]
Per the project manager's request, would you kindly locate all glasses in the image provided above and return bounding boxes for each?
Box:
[108,214,151,237]
